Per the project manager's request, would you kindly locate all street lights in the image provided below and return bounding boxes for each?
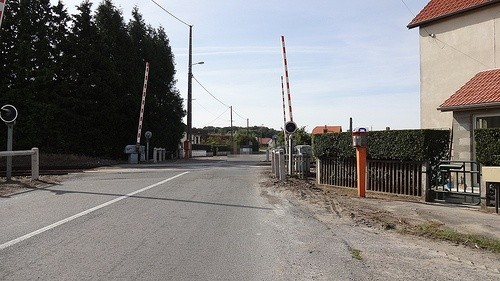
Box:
[186,61,205,159]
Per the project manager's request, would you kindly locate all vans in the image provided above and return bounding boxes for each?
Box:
[294,145,314,163]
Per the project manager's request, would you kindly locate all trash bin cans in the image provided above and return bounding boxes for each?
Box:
[294,144,311,173]
